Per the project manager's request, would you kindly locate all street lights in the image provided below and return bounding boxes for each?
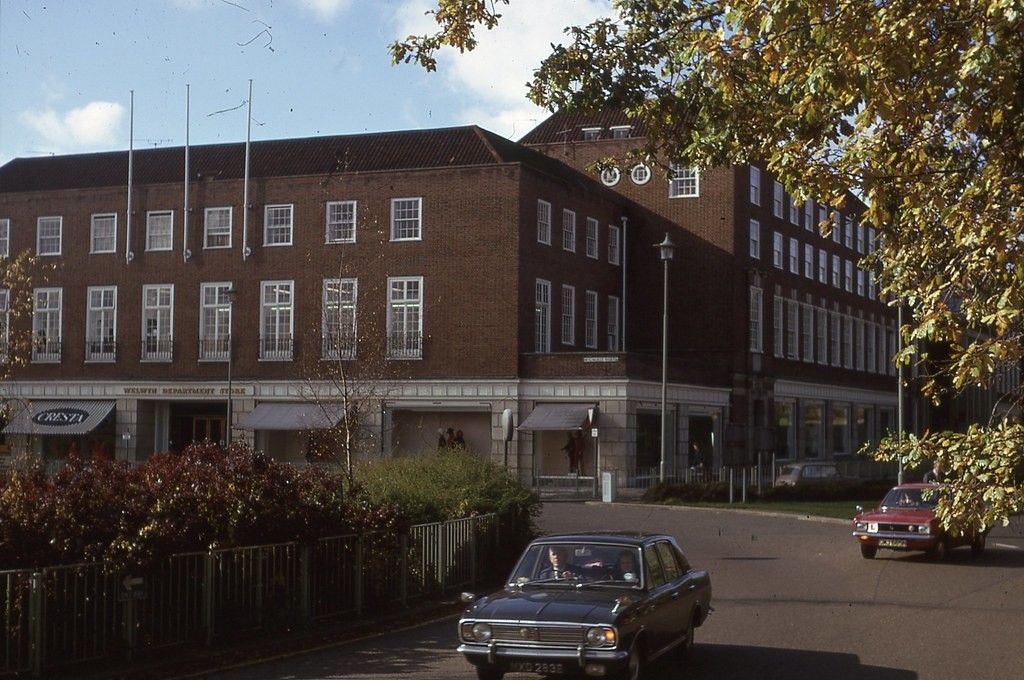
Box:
[652,229,678,482]
[887,298,905,486]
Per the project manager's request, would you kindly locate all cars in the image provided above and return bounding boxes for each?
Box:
[853,483,991,558]
[457,529,713,680]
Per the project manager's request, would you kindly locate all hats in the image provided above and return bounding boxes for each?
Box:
[437,428,444,435]
[457,429,464,434]
[446,428,454,433]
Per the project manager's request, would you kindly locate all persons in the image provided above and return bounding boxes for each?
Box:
[437,428,465,454]
[574,430,587,473]
[304,431,332,461]
[539,546,587,583]
[605,549,640,582]
[923,461,947,484]
[900,489,916,507]
[555,430,577,473]
[690,442,706,470]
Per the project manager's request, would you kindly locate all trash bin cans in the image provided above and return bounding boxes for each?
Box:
[601,466,618,503]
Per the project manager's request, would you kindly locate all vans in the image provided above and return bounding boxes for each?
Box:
[776,461,842,493]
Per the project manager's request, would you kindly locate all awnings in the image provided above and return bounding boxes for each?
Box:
[231,403,356,430]
[1,401,116,435]
[516,403,597,431]
